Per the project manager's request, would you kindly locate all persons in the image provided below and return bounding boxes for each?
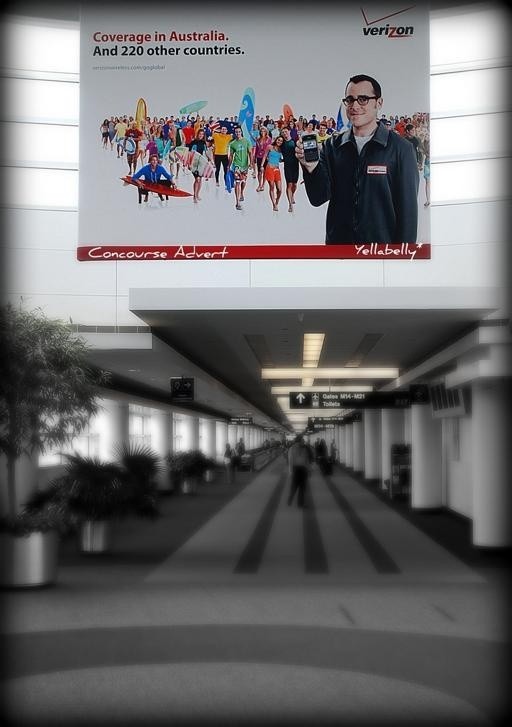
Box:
[286,433,313,509]
[292,73,422,247]
[97,111,432,213]
[224,443,236,487]
[235,437,245,455]
[260,436,338,482]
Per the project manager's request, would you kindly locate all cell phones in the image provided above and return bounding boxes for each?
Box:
[301,134,322,164]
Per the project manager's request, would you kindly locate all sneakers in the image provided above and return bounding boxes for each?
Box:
[256,186,264,192]
[157,193,164,201]
[236,204,241,210]
[239,194,244,201]
[193,196,201,203]
[273,198,295,212]
[144,193,148,202]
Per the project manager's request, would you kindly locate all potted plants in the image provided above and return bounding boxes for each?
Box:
[62,439,214,553]
[0,300,112,589]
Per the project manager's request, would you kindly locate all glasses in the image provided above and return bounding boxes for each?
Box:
[343,97,376,107]
